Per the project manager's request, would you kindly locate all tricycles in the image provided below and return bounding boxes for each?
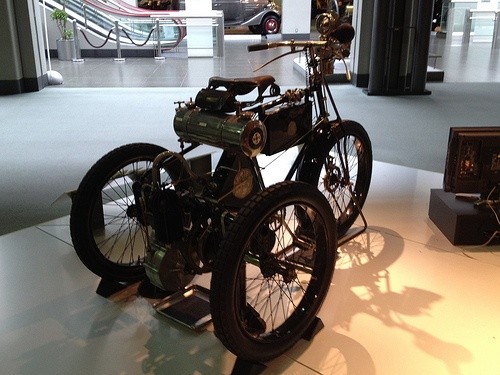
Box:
[68,8,374,360]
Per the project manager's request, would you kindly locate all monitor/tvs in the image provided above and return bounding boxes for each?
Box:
[443,126,500,210]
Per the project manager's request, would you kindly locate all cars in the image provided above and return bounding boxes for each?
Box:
[169,0,281,35]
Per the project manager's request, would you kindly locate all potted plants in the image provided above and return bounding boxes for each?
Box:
[50,7,82,61]
